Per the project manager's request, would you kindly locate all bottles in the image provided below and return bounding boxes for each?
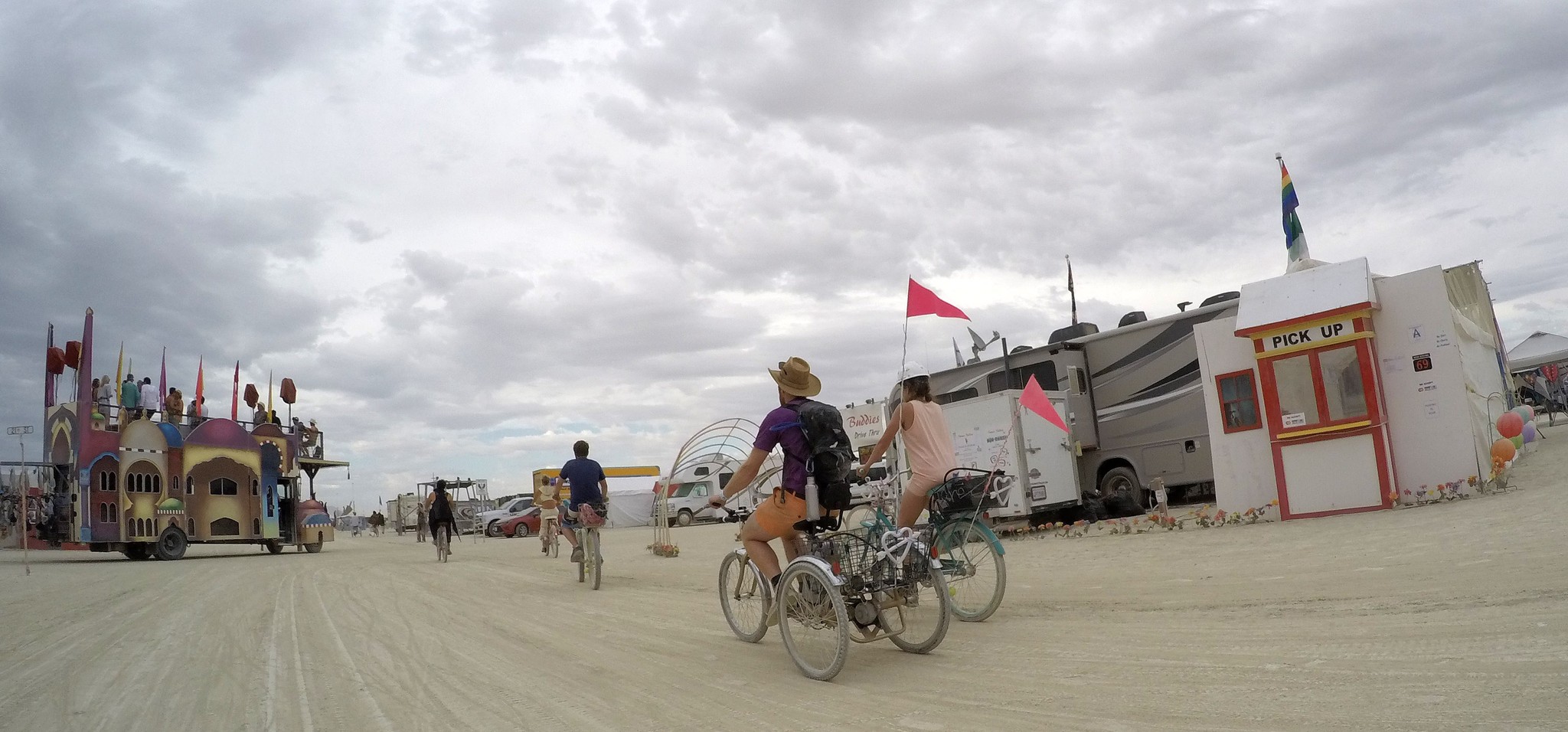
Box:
[951,474,960,494]
[805,477,819,522]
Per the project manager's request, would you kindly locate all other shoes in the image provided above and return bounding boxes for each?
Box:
[901,563,920,579]
[447,550,451,555]
[541,547,546,552]
[432,540,437,545]
[417,540,421,542]
[421,540,426,543]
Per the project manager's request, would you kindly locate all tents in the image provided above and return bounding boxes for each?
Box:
[1507,331,1568,420]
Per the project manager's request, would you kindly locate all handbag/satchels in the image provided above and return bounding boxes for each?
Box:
[928,475,1002,523]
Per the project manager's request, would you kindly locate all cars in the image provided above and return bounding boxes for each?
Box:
[492,503,566,538]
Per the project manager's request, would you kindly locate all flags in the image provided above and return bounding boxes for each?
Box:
[1288,208,1310,267]
[115,344,273,423]
[1281,159,1299,250]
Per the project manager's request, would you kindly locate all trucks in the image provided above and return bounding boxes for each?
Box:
[848,451,894,507]
[883,292,1242,505]
[653,456,784,528]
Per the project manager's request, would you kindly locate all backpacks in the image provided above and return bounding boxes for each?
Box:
[781,401,858,484]
[432,488,452,522]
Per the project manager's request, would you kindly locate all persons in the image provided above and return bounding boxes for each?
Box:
[425,481,455,554]
[531,476,561,552]
[349,512,359,534]
[856,360,958,608]
[91,373,209,429]
[707,357,846,627]
[371,511,378,532]
[416,502,426,542]
[552,440,608,565]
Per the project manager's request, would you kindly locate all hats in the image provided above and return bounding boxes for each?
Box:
[191,396,197,403]
[418,502,424,508]
[768,357,822,397]
[127,374,134,381]
[292,417,299,421]
[257,402,265,410]
[308,418,317,425]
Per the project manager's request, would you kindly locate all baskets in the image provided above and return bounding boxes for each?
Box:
[578,502,609,527]
[929,472,1014,514]
[791,523,935,593]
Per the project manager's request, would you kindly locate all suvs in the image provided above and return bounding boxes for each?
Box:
[474,496,535,538]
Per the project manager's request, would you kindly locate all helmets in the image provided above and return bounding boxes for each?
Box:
[894,360,930,386]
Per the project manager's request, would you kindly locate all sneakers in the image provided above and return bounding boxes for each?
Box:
[766,583,795,627]
[600,554,604,564]
[570,546,583,562]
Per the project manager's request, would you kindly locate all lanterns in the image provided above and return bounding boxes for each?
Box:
[1492,404,1537,462]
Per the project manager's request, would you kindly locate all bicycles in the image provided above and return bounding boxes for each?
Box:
[531,504,560,558]
[553,496,609,590]
[840,468,1007,623]
[425,510,452,563]
[369,525,379,537]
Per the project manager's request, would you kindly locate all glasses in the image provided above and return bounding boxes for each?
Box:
[779,362,790,375]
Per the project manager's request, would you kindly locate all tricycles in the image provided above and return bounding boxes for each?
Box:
[351,526,362,538]
[710,502,950,682]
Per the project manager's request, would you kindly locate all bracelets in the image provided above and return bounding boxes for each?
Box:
[720,490,731,501]
